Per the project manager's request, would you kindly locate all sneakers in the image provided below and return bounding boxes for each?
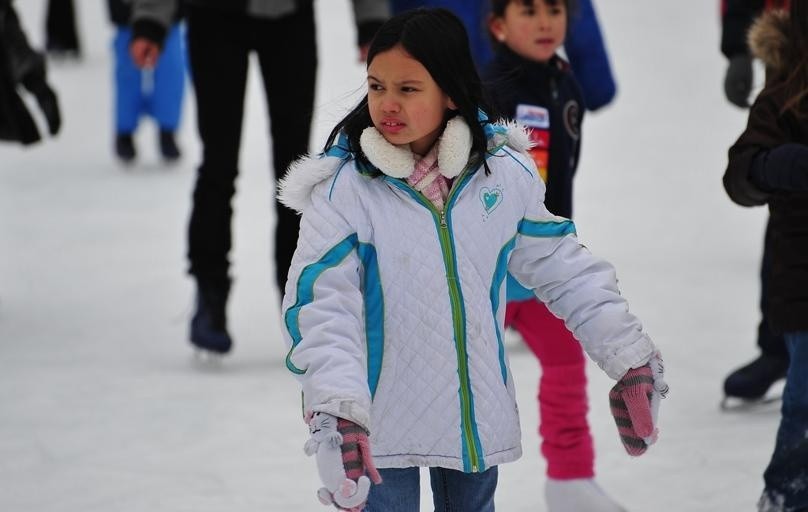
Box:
[187,287,234,354]
[113,131,181,163]
[722,355,787,402]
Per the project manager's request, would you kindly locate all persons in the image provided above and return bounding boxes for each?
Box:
[127,0,317,356]
[272,7,669,512]
[353,0,616,109]
[721,0,808,399]
[481,0,626,512]
[722,0,808,512]
[109,0,184,156]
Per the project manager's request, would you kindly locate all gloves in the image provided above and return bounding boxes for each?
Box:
[607,356,671,457]
[304,412,384,512]
